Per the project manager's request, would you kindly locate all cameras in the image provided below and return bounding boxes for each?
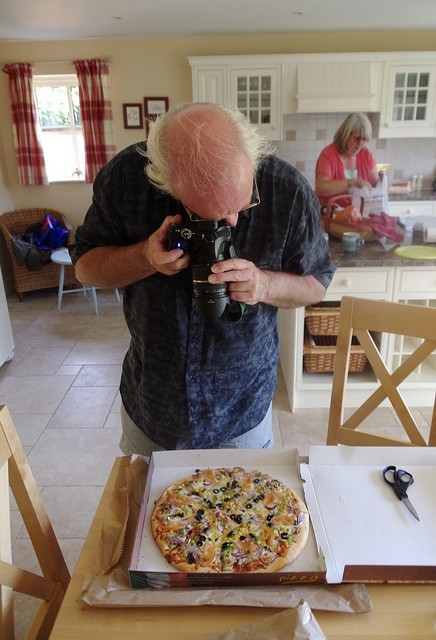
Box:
[167,215,246,325]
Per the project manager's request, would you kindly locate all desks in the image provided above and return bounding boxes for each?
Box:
[49,248,121,315]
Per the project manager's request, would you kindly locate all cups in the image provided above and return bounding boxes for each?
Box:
[341,231,365,252]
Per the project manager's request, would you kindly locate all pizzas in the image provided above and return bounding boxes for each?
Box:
[149,467,311,577]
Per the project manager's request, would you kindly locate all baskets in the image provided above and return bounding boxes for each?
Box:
[304,336,367,373]
[319,194,381,242]
[305,306,356,335]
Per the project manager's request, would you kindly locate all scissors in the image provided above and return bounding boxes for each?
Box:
[382,466,419,521]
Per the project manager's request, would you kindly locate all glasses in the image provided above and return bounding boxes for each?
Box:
[183,171,260,221]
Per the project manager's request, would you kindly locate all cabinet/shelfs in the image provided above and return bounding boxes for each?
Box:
[388,202,436,225]
[380,54,436,138]
[191,57,283,141]
[275,267,436,411]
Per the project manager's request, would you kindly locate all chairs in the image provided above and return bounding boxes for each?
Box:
[0,207,80,302]
[323,295,436,447]
[0,404,71,640]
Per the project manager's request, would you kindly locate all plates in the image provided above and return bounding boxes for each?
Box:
[394,245,436,260]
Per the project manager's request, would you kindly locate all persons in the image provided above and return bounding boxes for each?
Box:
[66,103,337,457]
[315,113,390,216]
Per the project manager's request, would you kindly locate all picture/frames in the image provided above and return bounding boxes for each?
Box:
[144,97,168,117]
[122,103,142,129]
